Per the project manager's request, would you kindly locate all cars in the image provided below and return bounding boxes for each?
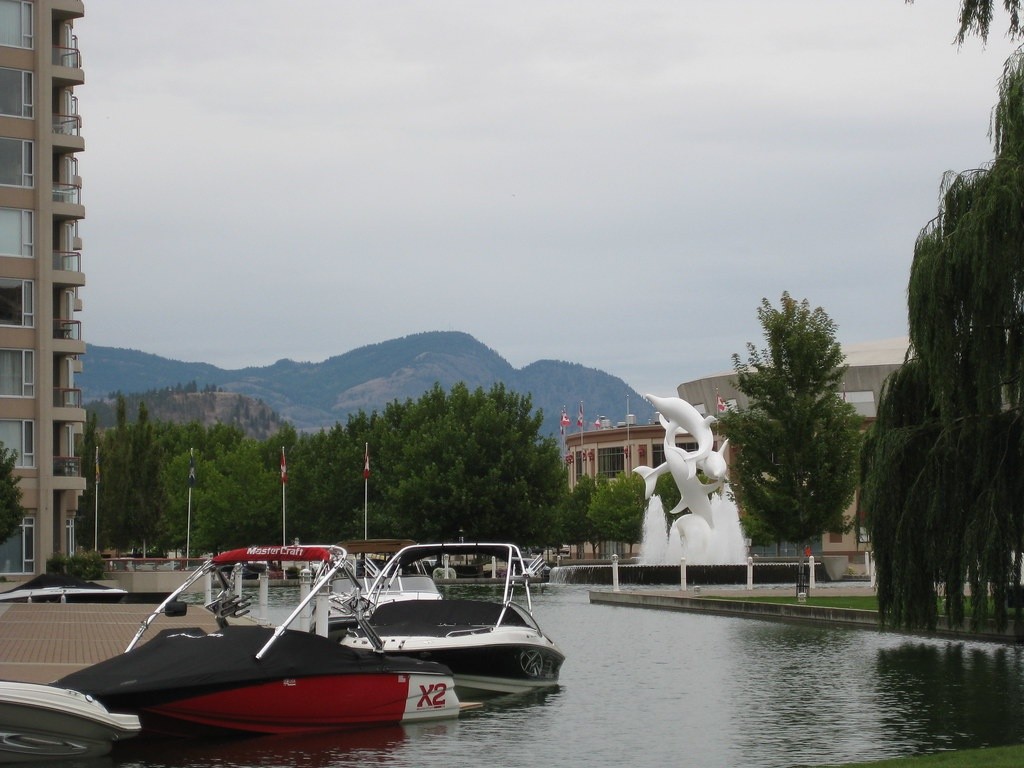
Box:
[110,557,264,582]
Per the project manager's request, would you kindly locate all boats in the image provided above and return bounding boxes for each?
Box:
[0,573,133,605]
[306,545,444,645]
[337,542,567,696]
[47,540,484,749]
[1,677,141,759]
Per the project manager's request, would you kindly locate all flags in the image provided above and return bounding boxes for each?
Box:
[188,449,196,488]
[559,410,564,436]
[362,444,370,479]
[716,391,725,411]
[593,417,601,427]
[561,406,571,427]
[843,386,849,404]
[577,403,584,428]
[280,447,287,483]
